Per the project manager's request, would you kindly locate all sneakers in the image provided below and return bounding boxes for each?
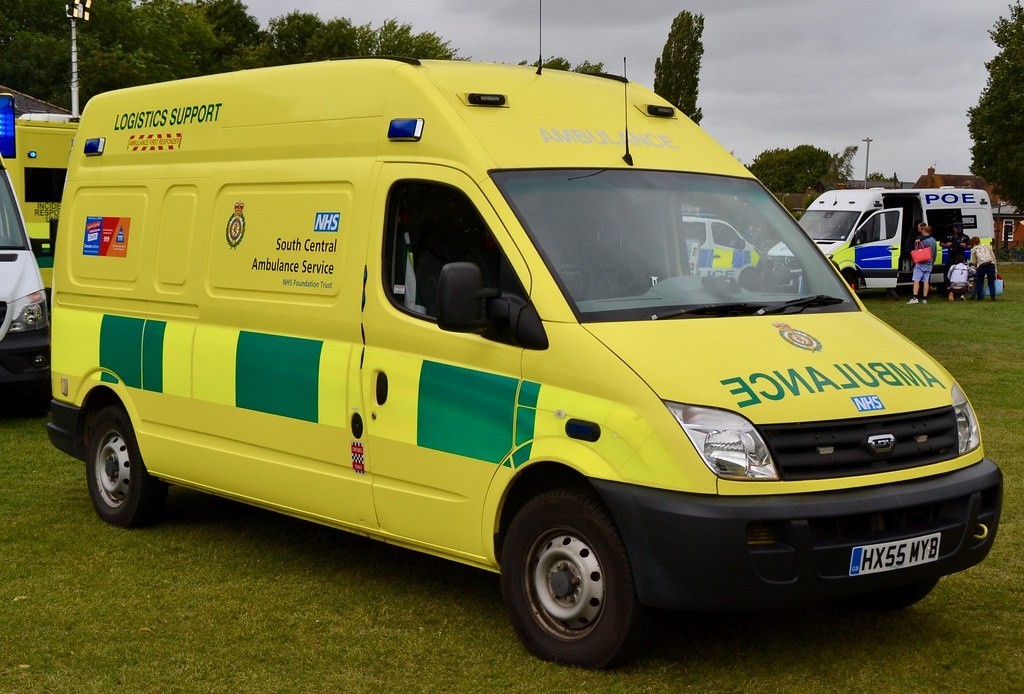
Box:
[922,300,927,303]
[907,298,918,304]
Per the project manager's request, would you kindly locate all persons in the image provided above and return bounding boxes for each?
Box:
[939,222,970,297]
[947,256,977,301]
[907,223,938,304]
[969,236,997,301]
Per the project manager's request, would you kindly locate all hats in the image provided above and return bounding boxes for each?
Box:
[953,222,964,229]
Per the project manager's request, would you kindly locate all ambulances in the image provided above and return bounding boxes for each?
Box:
[47,56,1004,672]
[4,114,81,254]
[0,92,51,411]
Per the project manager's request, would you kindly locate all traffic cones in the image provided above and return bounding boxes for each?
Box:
[997,274,1006,288]
[849,283,855,304]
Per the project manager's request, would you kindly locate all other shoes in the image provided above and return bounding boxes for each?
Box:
[960,295,967,301]
[949,292,954,301]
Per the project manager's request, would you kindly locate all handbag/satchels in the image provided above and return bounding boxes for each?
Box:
[986,274,1003,295]
[911,241,932,263]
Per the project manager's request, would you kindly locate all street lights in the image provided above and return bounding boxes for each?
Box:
[861,137,873,188]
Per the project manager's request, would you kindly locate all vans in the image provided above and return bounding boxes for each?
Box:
[682,212,763,291]
[768,186,998,297]
